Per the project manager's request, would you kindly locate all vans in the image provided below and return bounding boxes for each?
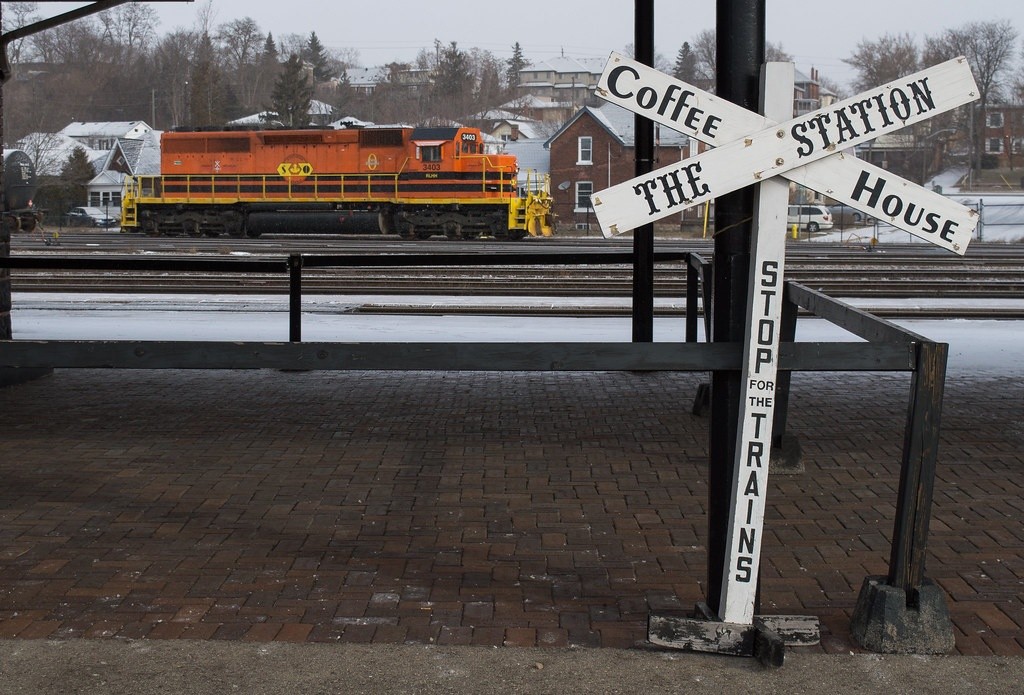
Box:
[787,204,834,232]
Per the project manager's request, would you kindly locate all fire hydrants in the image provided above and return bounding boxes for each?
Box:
[791,224,798,239]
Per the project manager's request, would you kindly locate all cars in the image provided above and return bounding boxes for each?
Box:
[825,202,875,225]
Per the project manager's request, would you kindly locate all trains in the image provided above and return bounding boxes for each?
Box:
[0,149,39,235]
[119,118,554,243]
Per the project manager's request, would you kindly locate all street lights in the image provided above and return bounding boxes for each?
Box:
[922,129,957,188]
